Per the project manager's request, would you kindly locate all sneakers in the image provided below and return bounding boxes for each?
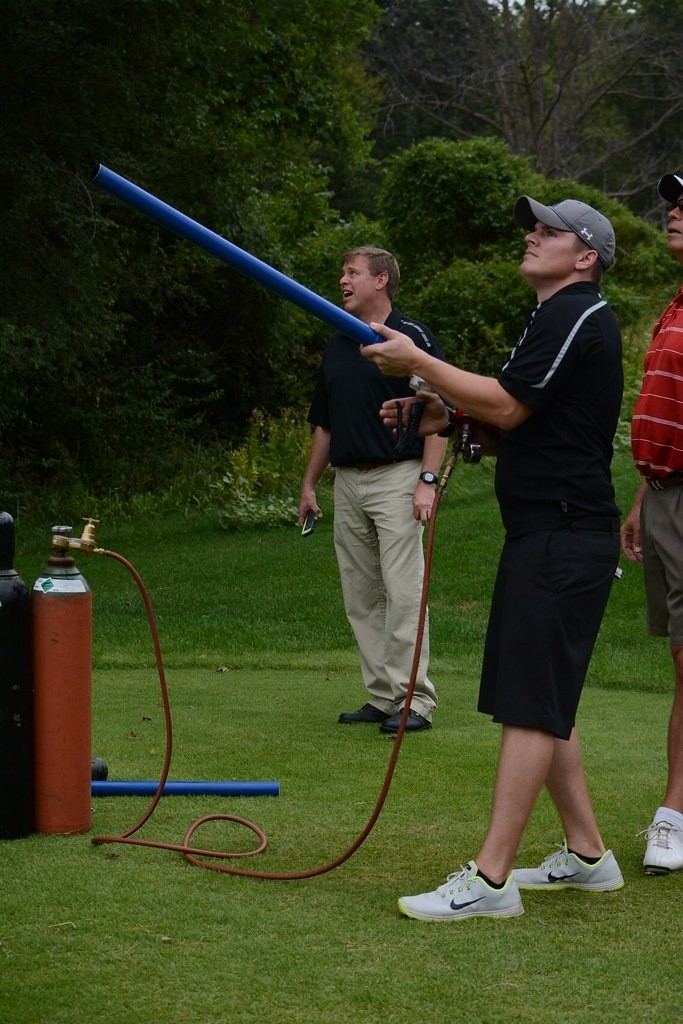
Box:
[635,819,683,875]
[337,703,393,724]
[379,707,432,733]
[513,838,625,892]
[398,860,524,921]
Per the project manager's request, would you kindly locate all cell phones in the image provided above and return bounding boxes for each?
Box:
[300,504,321,536]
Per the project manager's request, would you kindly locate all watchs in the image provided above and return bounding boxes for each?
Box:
[419,471,440,485]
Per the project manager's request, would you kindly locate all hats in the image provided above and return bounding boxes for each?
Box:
[657,173,683,203]
[513,196,616,272]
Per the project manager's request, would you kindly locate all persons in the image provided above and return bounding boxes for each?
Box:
[357,197,628,922]
[618,166,683,873]
[298,247,447,733]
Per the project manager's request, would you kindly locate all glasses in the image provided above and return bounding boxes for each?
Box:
[671,198,683,212]
